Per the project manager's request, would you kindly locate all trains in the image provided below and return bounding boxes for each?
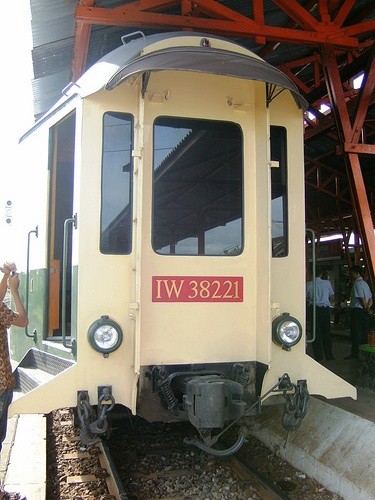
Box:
[7,29,356,460]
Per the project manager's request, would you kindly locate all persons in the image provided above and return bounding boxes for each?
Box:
[345,264,374,359]
[305,265,339,362]
[0,261,30,500]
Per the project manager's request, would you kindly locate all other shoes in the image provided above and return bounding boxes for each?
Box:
[325,351,335,360]
[344,352,359,360]
[0,489,27,500]
[314,352,322,360]
